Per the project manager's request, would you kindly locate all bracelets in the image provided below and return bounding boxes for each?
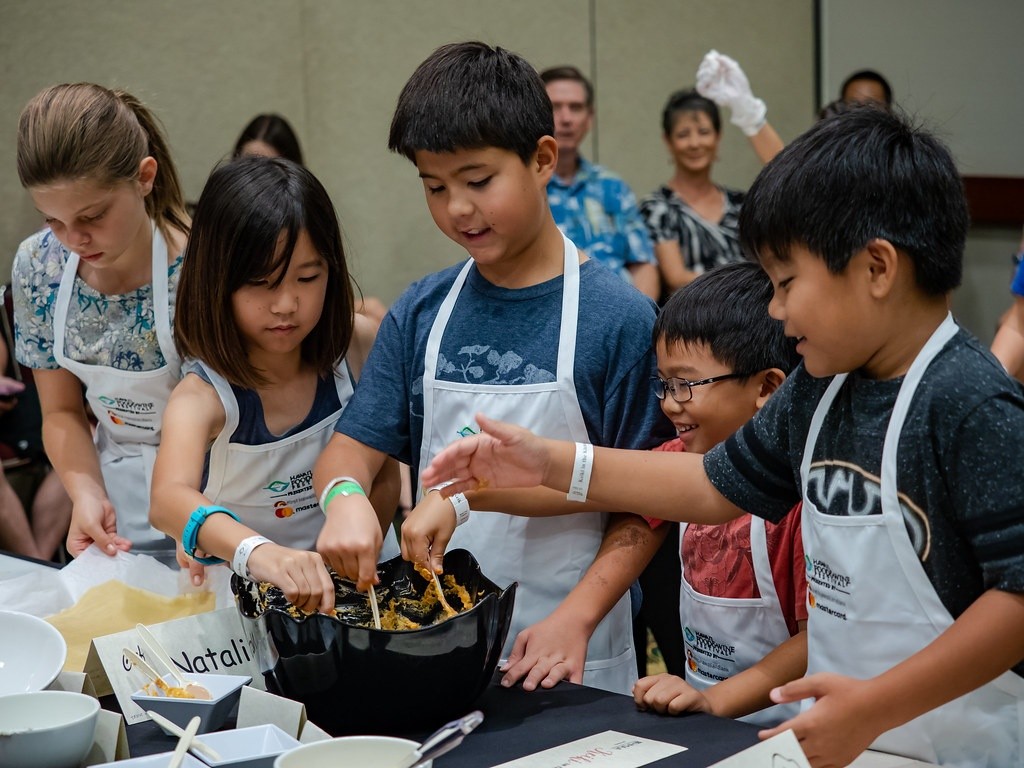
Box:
[181,505,239,565]
[232,535,273,583]
[743,118,767,137]
[318,476,365,515]
[567,443,594,503]
[427,480,469,526]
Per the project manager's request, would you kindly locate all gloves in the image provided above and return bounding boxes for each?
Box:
[696,50,767,136]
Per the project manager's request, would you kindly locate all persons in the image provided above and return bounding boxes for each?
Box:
[400,100,1024,768]
[990,243,1024,384]
[233,114,305,167]
[0,83,236,609]
[314,42,677,697]
[696,49,894,165]
[502,262,810,718]
[641,89,756,293]
[538,65,658,304]
[148,153,400,613]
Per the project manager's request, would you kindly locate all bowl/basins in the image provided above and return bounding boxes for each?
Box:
[188,723,301,768]
[129,672,254,736]
[230,548,519,744]
[0,690,101,768]
[273,734,434,768]
[0,609,68,698]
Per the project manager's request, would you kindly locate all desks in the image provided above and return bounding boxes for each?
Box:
[0,558,937,768]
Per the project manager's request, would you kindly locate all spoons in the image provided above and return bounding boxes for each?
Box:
[135,622,212,702]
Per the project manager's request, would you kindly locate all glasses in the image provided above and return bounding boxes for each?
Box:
[648,365,765,403]
